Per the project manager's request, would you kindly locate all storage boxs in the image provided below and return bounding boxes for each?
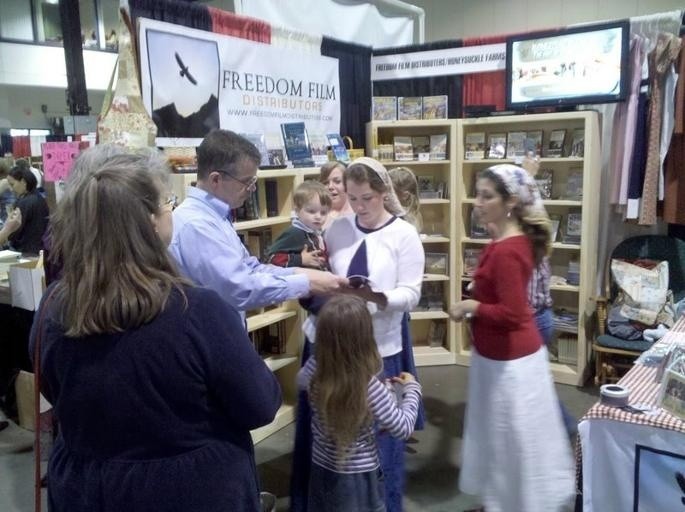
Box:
[14,369,35,432]
[7,261,44,310]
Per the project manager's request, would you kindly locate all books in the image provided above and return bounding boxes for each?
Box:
[465,129,566,159]
[426,134,449,348]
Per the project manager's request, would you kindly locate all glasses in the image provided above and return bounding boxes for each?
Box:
[216,170,258,191]
[158,193,177,212]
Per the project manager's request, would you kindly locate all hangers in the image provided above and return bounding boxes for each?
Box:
[626,9,685,48]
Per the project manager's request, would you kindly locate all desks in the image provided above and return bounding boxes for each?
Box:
[0,256,45,311]
[574,310,685,512]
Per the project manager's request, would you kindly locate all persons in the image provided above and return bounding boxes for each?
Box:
[451,163,577,512]
[1,128,426,512]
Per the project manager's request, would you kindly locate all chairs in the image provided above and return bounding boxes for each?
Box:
[592,232,685,389]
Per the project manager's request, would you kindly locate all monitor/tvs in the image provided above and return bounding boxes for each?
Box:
[505,18,630,112]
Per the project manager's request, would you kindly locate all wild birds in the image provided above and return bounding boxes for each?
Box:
[173,51,198,86]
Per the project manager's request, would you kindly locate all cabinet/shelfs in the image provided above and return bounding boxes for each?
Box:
[169,167,302,447]
[302,167,323,181]
[371,118,456,368]
[456,110,603,386]
